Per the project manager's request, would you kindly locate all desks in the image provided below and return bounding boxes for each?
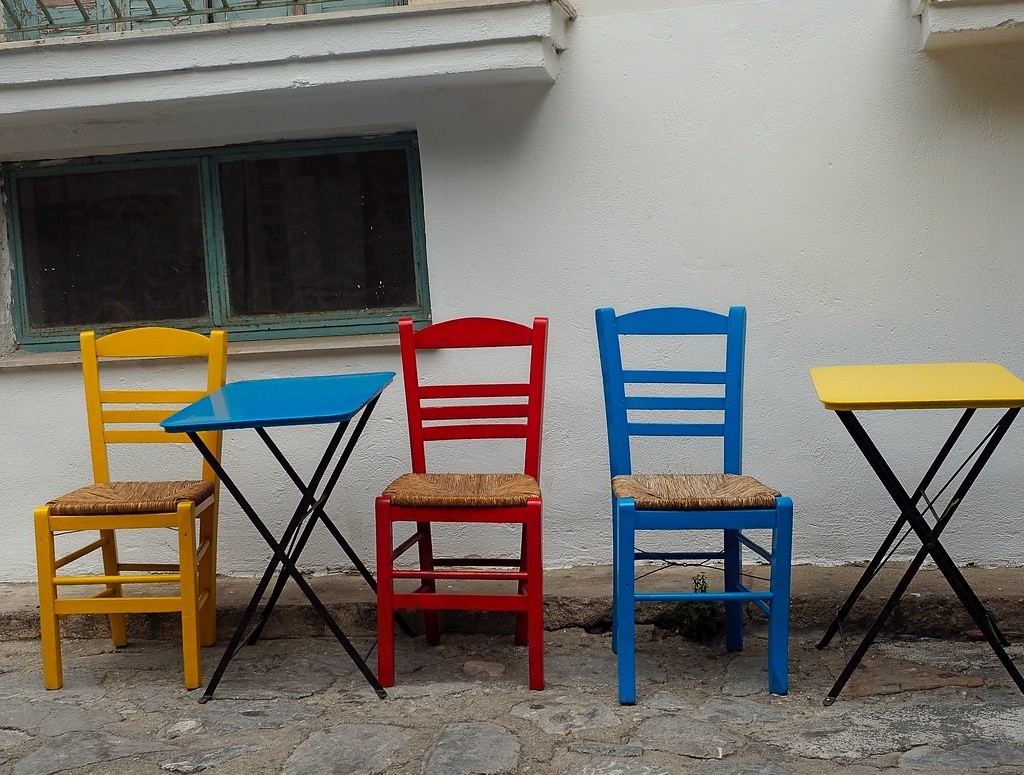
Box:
[809,363,1024,705]
[160,371,388,704]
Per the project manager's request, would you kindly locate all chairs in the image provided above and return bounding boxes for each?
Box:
[34,327,227,690]
[375,316,550,691]
[594,304,794,704]
